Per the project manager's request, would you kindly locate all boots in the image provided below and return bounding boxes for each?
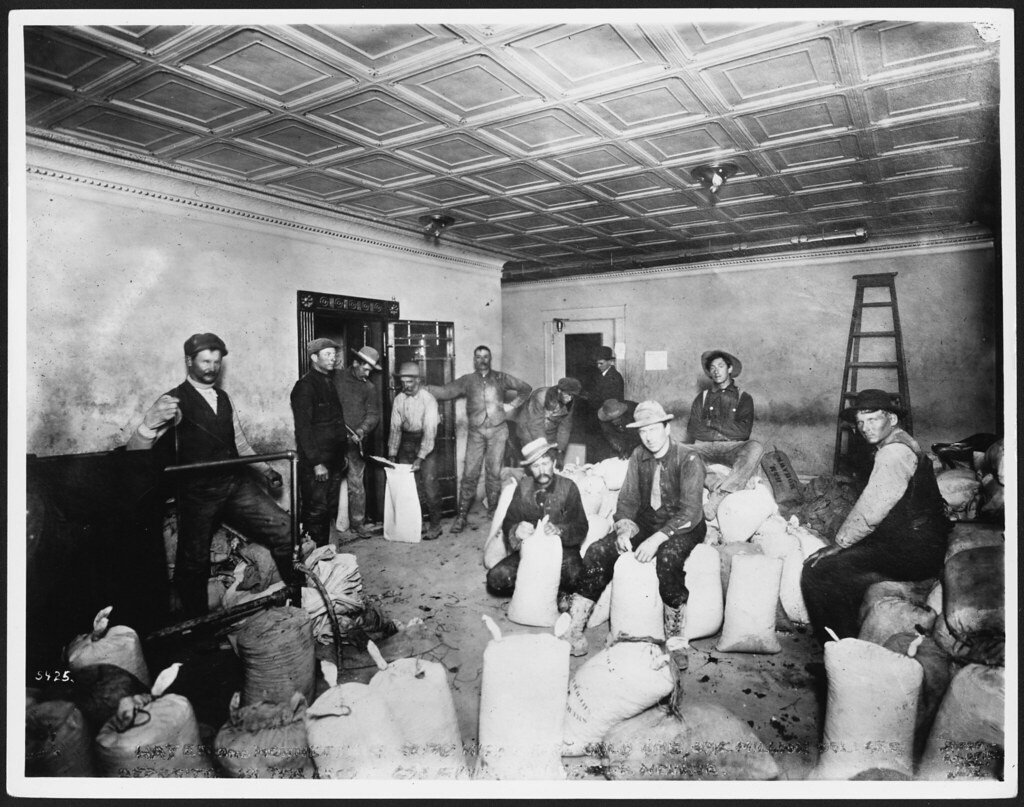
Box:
[663,601,688,669]
[564,594,595,656]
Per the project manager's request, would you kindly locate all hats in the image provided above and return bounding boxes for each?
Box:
[558,378,589,399]
[308,339,341,354]
[351,346,382,370]
[702,351,742,379]
[184,333,227,357]
[393,363,422,377]
[625,400,674,427]
[518,437,558,466]
[598,399,627,421]
[839,389,908,424]
[587,346,616,360]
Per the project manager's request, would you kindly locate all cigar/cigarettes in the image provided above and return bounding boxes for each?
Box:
[363,378,366,382]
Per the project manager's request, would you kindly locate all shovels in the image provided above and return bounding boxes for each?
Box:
[344,422,394,468]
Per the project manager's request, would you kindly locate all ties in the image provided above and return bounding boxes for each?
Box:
[650,464,661,511]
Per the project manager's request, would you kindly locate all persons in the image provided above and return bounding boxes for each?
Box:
[424,346,532,533]
[485,437,589,595]
[515,346,625,462]
[799,389,945,643]
[127,332,307,655]
[564,400,706,670]
[388,362,443,540]
[290,338,346,563]
[330,346,383,553]
[683,350,764,521]
[597,400,643,460]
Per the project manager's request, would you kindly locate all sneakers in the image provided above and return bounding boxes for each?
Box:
[423,526,443,541]
[351,520,370,537]
[703,490,735,518]
[449,517,467,533]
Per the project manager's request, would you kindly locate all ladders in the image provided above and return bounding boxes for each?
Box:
[834,271,914,475]
